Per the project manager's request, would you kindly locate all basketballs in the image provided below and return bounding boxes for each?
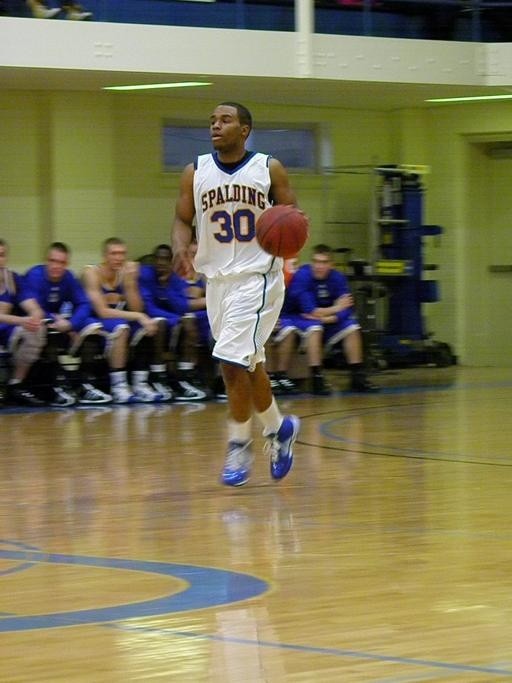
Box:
[254,204,308,259]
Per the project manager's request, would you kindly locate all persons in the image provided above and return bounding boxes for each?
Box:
[268,251,303,394]
[181,237,229,398]
[138,243,208,401]
[0,237,49,407]
[16,240,113,407]
[286,241,380,395]
[166,100,311,485]
[78,236,164,405]
[207,490,292,682]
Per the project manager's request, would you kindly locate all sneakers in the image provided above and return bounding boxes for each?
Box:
[10,381,44,406]
[133,378,224,402]
[268,373,380,395]
[50,380,77,408]
[222,439,258,487]
[79,382,111,404]
[262,414,300,482]
[111,385,140,404]
